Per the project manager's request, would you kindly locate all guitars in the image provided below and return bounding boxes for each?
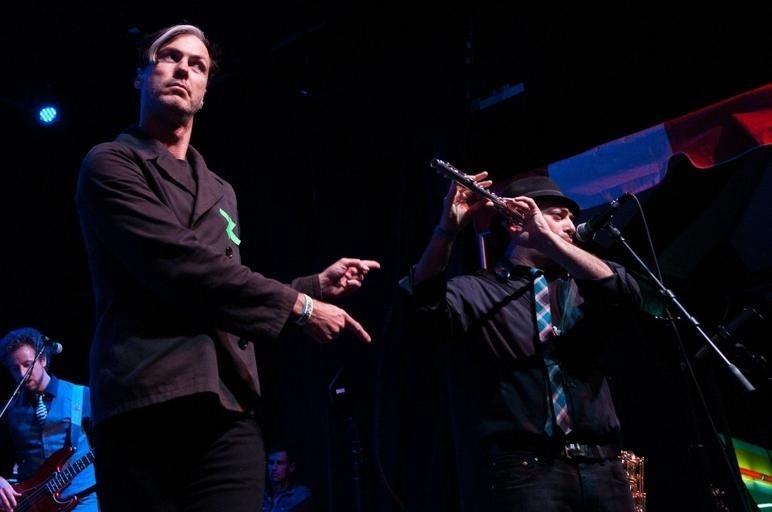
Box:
[0,445,98,512]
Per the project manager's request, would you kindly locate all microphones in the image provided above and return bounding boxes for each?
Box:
[575,193,627,243]
[41,334,63,355]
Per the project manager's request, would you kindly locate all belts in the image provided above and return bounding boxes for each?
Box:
[503,439,621,461]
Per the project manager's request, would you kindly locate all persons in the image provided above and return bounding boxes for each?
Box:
[74,23,389,511]
[258,442,314,511]
[397,172,647,512]
[0,328,103,511]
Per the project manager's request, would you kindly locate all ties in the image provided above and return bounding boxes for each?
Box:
[528,267,575,440]
[35,394,48,426]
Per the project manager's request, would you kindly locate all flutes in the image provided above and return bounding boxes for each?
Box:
[430,157,528,228]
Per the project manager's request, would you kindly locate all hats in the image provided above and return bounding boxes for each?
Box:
[490,176,582,244]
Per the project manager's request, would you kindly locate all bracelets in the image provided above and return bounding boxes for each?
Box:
[293,292,313,327]
[431,224,460,244]
